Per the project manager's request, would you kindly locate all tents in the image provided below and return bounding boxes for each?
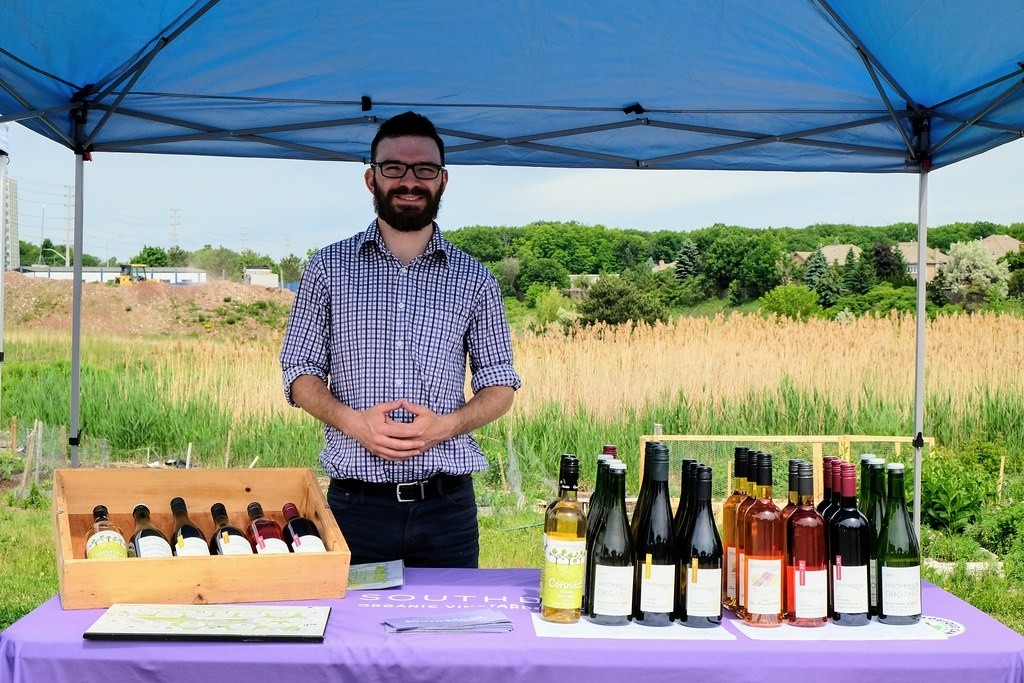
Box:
[0,0,1024,555]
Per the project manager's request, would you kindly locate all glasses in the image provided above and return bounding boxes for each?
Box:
[371,160,445,180]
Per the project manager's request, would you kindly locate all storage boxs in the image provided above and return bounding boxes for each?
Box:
[51,466,351,612]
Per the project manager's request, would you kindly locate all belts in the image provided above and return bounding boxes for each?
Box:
[330,473,472,502]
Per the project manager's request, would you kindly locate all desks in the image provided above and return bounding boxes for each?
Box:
[0,566,1024,683]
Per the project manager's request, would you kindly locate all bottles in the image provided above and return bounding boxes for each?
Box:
[283,503,328,552]
[84,506,126,558]
[245,502,289,554]
[170,497,209,556]
[210,502,254,554]
[540,441,924,628]
[131,503,172,557]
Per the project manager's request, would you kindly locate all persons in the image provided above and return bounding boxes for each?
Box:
[283,110,519,566]
